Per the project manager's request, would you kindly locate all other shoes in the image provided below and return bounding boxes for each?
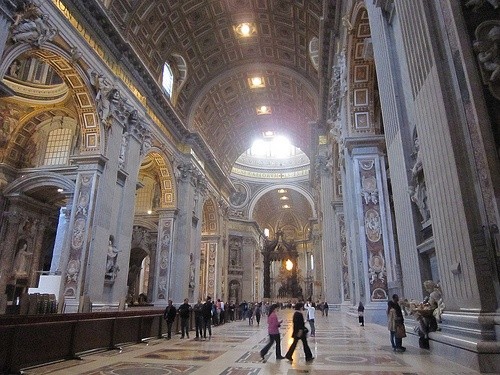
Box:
[260,352,265,360]
[277,356,285,359]
[305,357,315,361]
[285,355,293,361]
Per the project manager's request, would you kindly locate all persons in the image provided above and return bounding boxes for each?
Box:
[357,302,368,327]
[382,293,408,352]
[413,309,439,349]
[302,299,319,338]
[164,299,177,340]
[178,296,192,341]
[202,296,214,339]
[259,303,285,362]
[194,296,205,341]
[285,303,316,363]
[265,297,330,317]
[212,298,262,327]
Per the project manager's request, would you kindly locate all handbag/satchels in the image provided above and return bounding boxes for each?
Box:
[397,325,406,338]
